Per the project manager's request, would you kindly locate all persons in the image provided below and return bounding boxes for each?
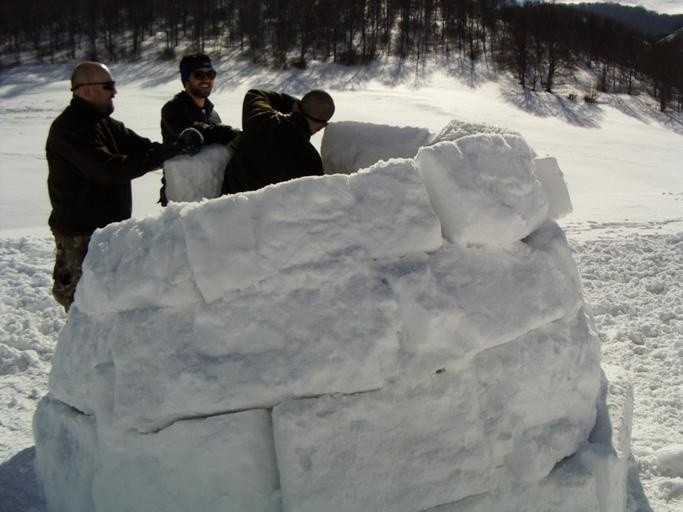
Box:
[220,87,334,197]
[41,60,204,319]
[155,54,244,207]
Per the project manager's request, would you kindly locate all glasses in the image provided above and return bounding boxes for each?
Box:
[192,70,216,80]
[71,82,116,88]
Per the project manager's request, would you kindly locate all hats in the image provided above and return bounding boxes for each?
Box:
[179,52,211,85]
